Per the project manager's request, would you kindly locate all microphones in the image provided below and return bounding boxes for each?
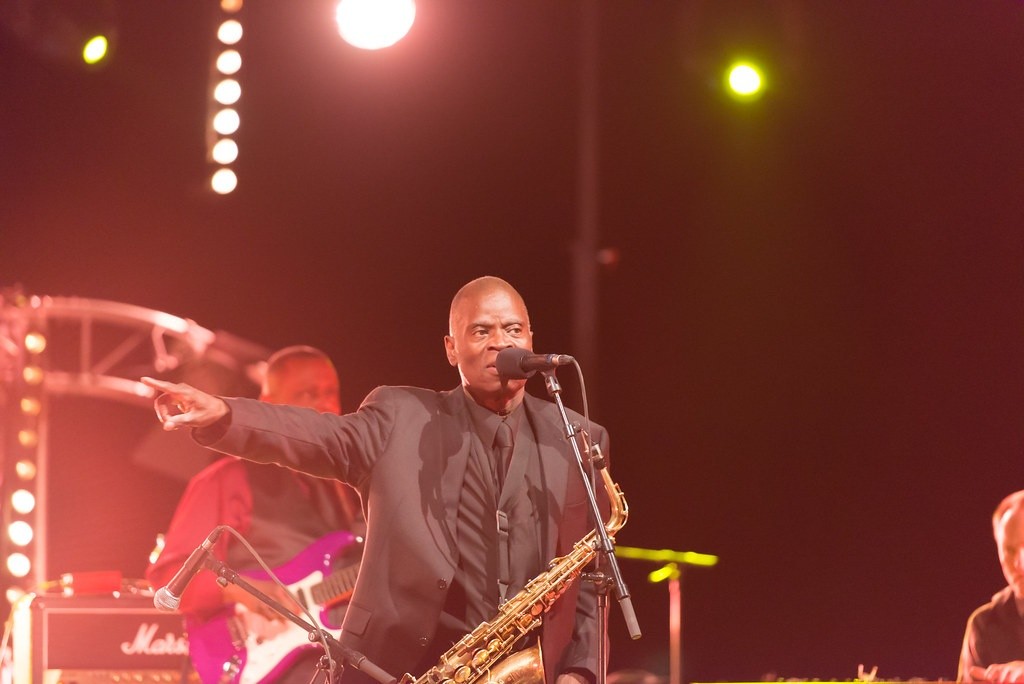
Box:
[153,527,221,611]
[495,347,573,379]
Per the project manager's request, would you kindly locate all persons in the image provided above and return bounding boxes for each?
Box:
[140,277,613,684]
[146,344,359,684]
[955,488,1024,684]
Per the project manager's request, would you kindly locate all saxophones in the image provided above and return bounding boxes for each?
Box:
[400,422,629,684]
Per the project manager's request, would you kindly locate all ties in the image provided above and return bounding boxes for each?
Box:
[495,422,513,494]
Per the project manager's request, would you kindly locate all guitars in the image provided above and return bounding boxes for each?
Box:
[186,528,358,684]
